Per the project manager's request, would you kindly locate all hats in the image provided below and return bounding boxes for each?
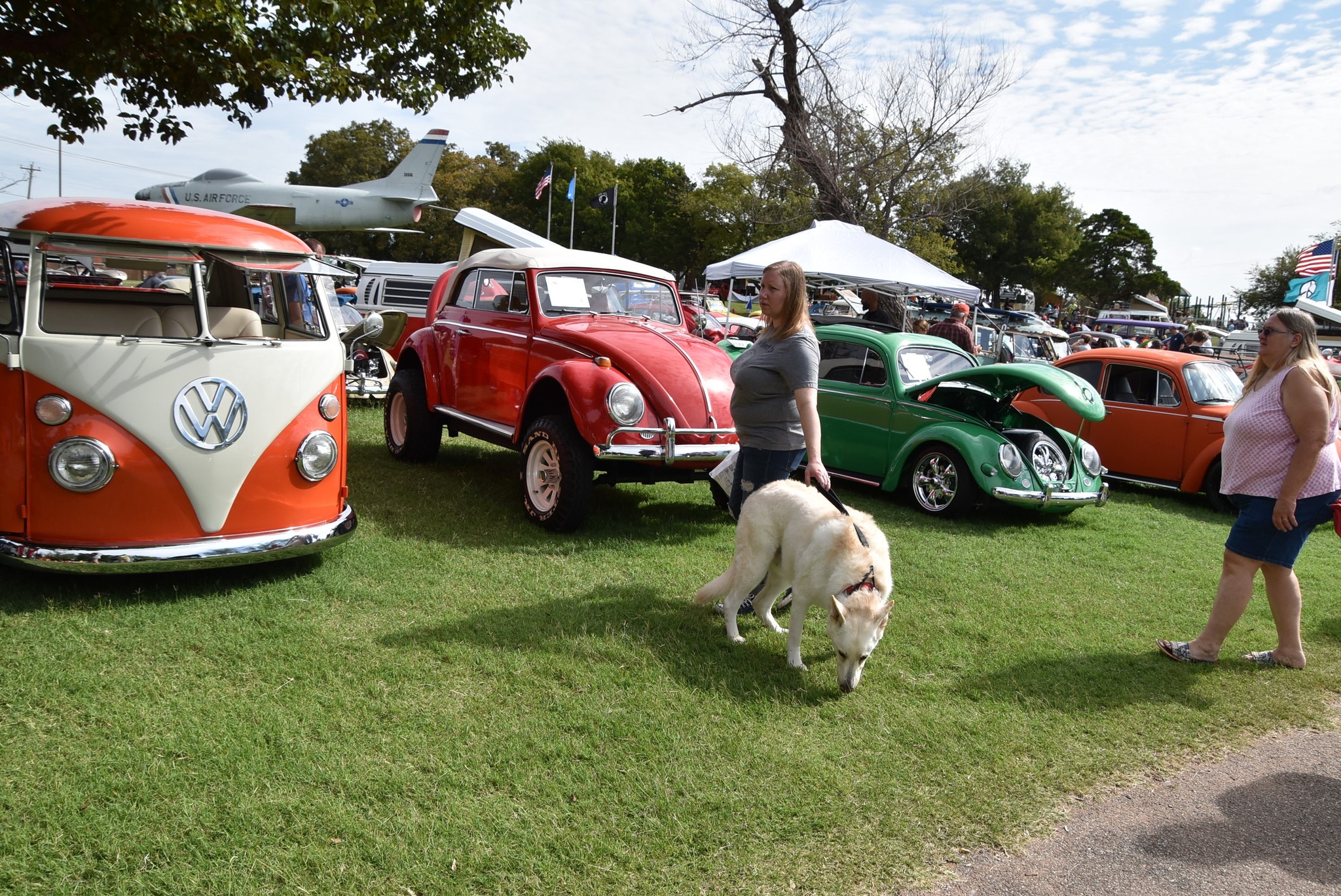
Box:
[951,303,972,319]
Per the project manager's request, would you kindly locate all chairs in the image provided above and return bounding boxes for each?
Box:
[161,305,263,340]
[1104,372,1139,404]
[1144,376,1178,406]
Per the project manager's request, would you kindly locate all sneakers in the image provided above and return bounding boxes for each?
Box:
[714,587,794,618]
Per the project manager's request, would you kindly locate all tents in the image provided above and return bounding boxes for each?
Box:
[702,219,981,347]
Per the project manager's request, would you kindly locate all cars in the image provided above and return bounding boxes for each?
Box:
[620,279,1341,382]
[715,323,1110,520]
[330,300,409,410]
[385,248,742,534]
[1011,348,1341,509]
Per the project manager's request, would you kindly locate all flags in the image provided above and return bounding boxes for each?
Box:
[1299,250,1339,277]
[1283,271,1329,302]
[590,188,614,209]
[565,177,575,202]
[1295,239,1333,274]
[535,166,551,200]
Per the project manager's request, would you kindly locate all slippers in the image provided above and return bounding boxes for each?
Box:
[1155,638,1304,671]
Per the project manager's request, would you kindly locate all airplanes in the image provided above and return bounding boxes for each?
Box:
[135,130,450,233]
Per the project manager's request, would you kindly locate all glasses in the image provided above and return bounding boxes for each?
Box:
[1258,329,1295,336]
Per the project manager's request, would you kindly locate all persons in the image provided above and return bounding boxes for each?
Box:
[714,260,832,614]
[926,303,983,358]
[14,260,29,278]
[860,289,892,327]
[282,238,326,330]
[1154,307,1341,670]
[985,294,1249,408]
[912,319,929,335]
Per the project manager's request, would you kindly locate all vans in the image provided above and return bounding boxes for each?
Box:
[0,206,579,320]
[0,194,384,577]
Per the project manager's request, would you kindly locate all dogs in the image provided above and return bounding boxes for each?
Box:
[694,478,895,699]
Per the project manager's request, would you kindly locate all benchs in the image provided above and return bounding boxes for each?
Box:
[1,296,161,337]
